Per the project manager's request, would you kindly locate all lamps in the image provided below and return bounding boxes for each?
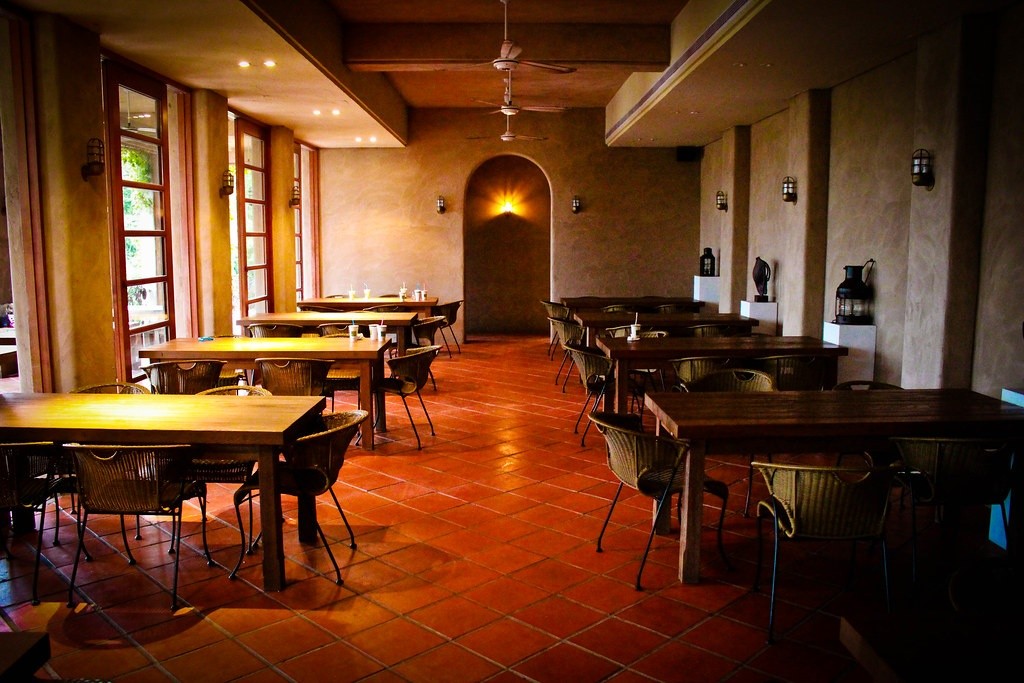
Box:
[571,195,582,214]
[911,147,935,191]
[436,195,446,215]
[501,202,514,218]
[289,186,300,208]
[80,137,105,182]
[218,170,234,199]
[715,190,729,213]
[780,176,798,206]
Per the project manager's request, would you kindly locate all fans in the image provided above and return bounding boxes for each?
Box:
[440,0,577,74]
[466,115,550,142]
[471,70,566,116]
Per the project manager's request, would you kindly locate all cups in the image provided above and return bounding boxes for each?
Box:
[630,324,641,340]
[399,292,405,302]
[348,324,359,342]
[349,291,355,301]
[414,290,421,301]
[364,289,370,299]
[377,325,387,341]
[368,324,379,340]
[421,290,427,300]
[401,288,407,298]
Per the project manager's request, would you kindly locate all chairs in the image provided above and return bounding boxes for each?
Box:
[0,287,465,614]
[538,295,1024,652]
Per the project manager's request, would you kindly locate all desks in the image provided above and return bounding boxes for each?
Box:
[297,295,439,319]
[642,387,1023,588]
[0,392,329,596]
[561,297,705,309]
[240,311,419,355]
[573,312,760,348]
[134,334,397,464]
[596,335,849,417]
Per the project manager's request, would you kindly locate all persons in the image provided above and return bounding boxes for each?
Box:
[5,303,15,328]
